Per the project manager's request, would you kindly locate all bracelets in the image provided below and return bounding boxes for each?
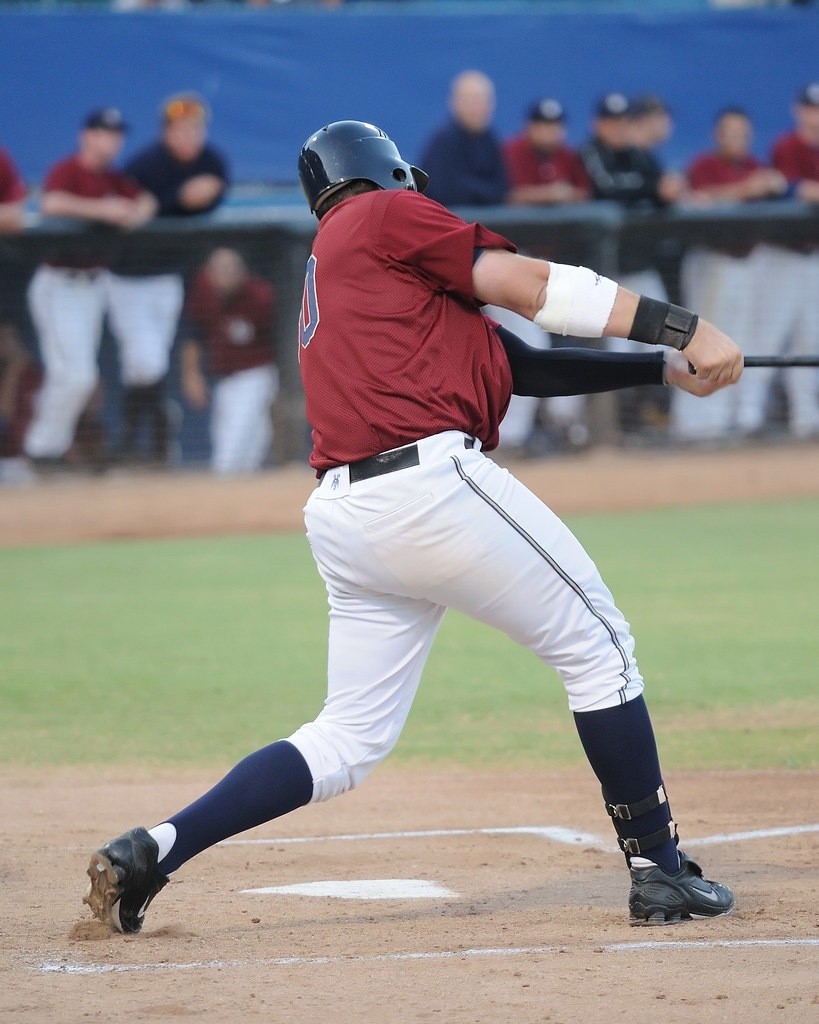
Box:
[628,294,699,351]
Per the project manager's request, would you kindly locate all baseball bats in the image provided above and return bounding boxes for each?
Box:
[688,354,819,375]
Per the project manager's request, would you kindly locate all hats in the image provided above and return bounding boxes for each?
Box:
[596,93,639,116]
[527,99,564,122]
[797,82,818,108]
[85,107,126,130]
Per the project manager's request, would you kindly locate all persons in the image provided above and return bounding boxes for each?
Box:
[413,76,819,451]
[83,120,744,934]
[0,92,281,482]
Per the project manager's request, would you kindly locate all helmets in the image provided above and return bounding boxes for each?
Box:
[298,120,430,214]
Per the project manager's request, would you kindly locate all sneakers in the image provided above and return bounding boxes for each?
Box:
[628,849,735,926]
[81,828,170,934]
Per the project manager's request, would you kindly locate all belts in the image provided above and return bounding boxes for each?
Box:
[318,438,474,487]
